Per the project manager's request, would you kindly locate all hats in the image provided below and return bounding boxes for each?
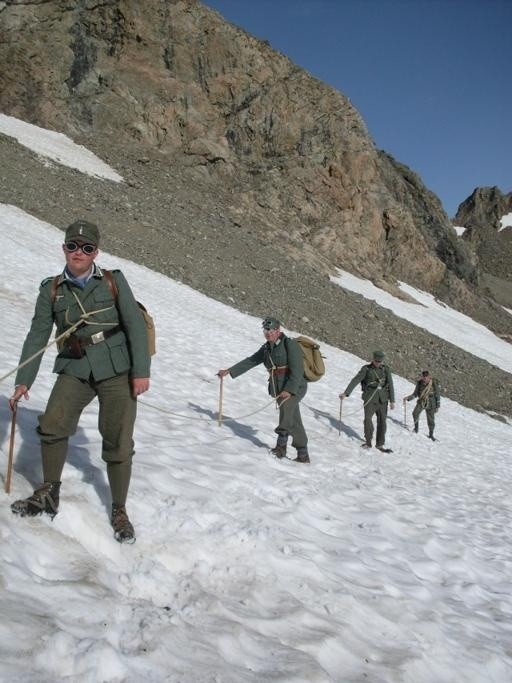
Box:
[260,316,281,329]
[64,219,102,245]
[421,371,430,377]
[372,351,386,359]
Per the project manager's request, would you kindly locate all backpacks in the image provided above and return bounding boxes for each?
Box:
[51,273,156,356]
[284,335,325,382]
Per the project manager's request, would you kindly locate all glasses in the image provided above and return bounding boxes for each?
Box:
[65,242,99,255]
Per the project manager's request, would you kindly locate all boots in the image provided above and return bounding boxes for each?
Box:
[13,481,62,513]
[293,447,311,464]
[109,503,135,541]
[412,421,437,441]
[362,438,393,453]
[270,445,286,458]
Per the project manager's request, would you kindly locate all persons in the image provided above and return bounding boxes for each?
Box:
[340,350,395,454]
[404,369,440,441]
[215,316,311,462]
[10,219,150,542]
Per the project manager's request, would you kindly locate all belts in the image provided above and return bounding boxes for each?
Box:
[60,324,125,348]
[369,385,386,390]
